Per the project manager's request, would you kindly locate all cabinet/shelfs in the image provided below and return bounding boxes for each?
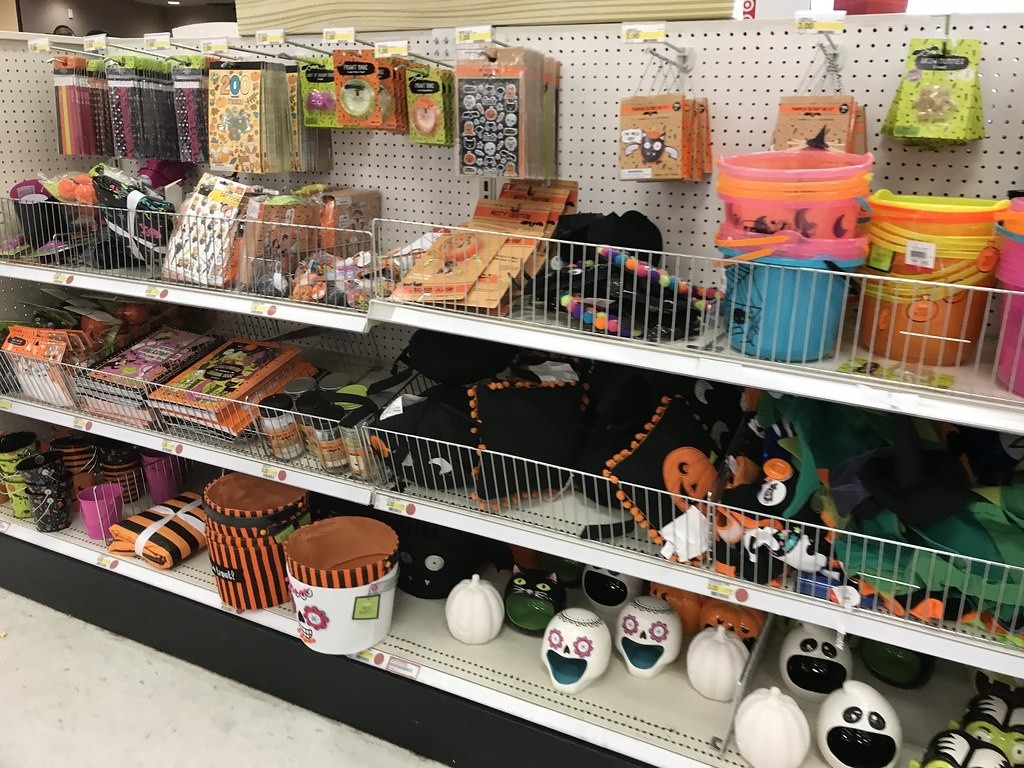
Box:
[0,259,1024,768]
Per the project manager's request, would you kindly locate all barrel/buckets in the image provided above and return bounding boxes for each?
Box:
[199,470,401,654]
[1,423,187,542]
[713,150,1023,396]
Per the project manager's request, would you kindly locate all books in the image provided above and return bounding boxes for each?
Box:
[147,338,331,443]
[69,326,222,433]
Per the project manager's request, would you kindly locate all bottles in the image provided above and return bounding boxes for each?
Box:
[260,373,398,481]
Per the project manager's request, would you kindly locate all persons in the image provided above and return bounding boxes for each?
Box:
[53,25,76,36]
